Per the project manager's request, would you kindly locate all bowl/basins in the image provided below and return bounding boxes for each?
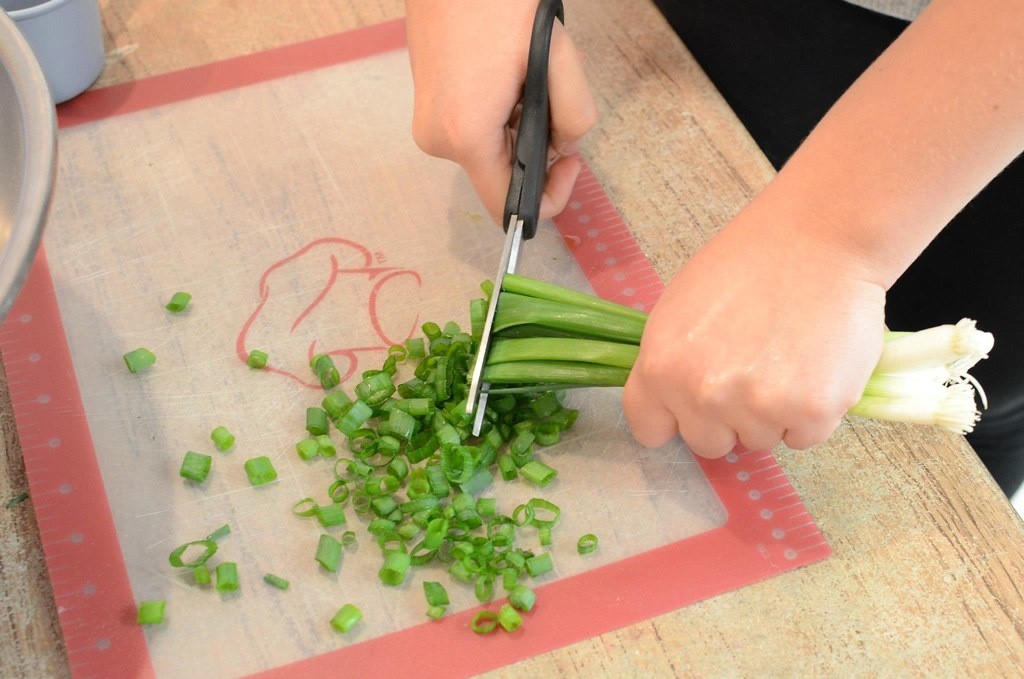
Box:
[0,5,58,325]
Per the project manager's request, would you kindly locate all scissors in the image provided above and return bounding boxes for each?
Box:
[463,0,566,439]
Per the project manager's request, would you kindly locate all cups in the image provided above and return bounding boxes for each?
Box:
[0,0,105,105]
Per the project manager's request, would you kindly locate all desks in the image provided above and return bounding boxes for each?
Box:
[0,0,1024,679]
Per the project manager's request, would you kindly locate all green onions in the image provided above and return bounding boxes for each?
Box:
[123,272,995,632]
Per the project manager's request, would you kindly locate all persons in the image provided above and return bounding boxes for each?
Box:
[404,0,1024,499]
